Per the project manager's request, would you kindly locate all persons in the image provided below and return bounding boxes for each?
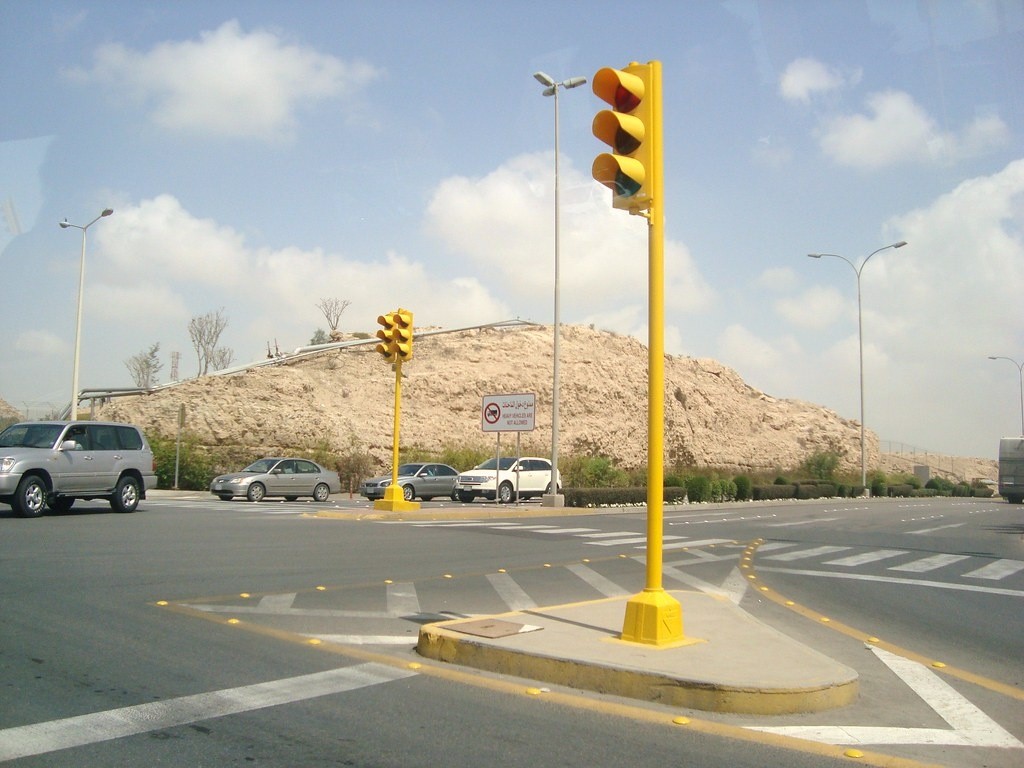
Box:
[65,431,83,450]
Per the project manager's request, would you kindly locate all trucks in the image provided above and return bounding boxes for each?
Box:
[998,438,1024,501]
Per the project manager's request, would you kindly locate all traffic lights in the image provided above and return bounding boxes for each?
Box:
[392,309,412,362]
[592,63,653,210]
[377,313,396,364]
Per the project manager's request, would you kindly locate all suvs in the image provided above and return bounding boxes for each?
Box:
[454,458,563,503]
[0,421,159,518]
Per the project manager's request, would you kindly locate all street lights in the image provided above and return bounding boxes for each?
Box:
[989,355,1024,435]
[532,70,586,509]
[60,208,115,422]
[806,242,908,485]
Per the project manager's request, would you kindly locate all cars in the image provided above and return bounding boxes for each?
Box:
[360,462,460,502]
[211,456,341,502]
